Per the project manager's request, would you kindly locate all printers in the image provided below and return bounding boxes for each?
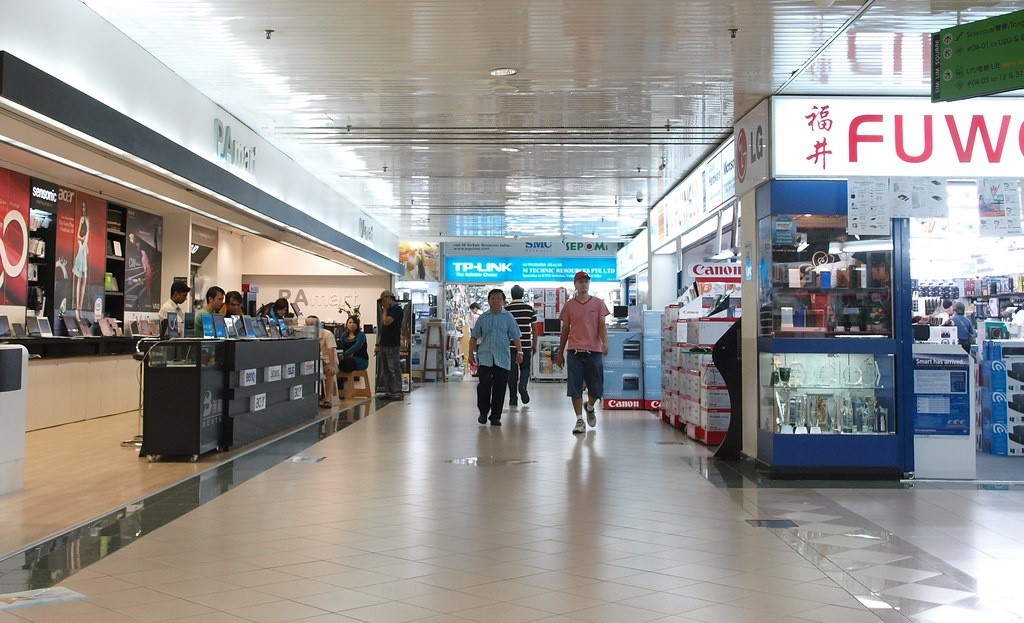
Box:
[622,375,639,390]
[622,335,640,359]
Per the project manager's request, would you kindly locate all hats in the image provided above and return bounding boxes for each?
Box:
[574,271,591,283]
[377,290,395,303]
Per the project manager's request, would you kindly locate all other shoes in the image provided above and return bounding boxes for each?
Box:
[378,392,393,400]
[478,411,487,424]
[339,396,345,401]
[519,384,530,404]
[323,399,332,409]
[392,392,403,401]
[509,399,517,406]
[472,373,479,377]
[491,417,501,426]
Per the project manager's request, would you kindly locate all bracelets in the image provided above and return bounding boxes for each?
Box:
[517,351,524,356]
[375,343,379,347]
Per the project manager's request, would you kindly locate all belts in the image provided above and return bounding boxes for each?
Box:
[568,348,602,356]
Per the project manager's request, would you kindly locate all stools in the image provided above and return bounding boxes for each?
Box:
[335,370,371,401]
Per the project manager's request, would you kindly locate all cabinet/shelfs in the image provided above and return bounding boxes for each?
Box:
[912,289,1024,339]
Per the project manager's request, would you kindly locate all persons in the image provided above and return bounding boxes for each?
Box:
[337,316,368,401]
[71,200,90,317]
[218,290,245,318]
[265,296,289,319]
[504,285,537,405]
[157,281,192,336]
[194,286,225,340]
[373,291,405,401]
[469,289,524,425]
[934,299,1015,355]
[469,303,484,377]
[558,272,610,433]
[307,315,340,408]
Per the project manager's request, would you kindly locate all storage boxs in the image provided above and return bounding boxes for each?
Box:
[661,304,731,444]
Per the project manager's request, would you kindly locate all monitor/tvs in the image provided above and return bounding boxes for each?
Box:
[543,318,562,335]
[613,306,628,319]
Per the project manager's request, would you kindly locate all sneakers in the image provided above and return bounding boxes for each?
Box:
[573,419,586,433]
[584,401,597,427]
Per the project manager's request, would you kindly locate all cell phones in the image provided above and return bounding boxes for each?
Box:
[0,312,289,339]
